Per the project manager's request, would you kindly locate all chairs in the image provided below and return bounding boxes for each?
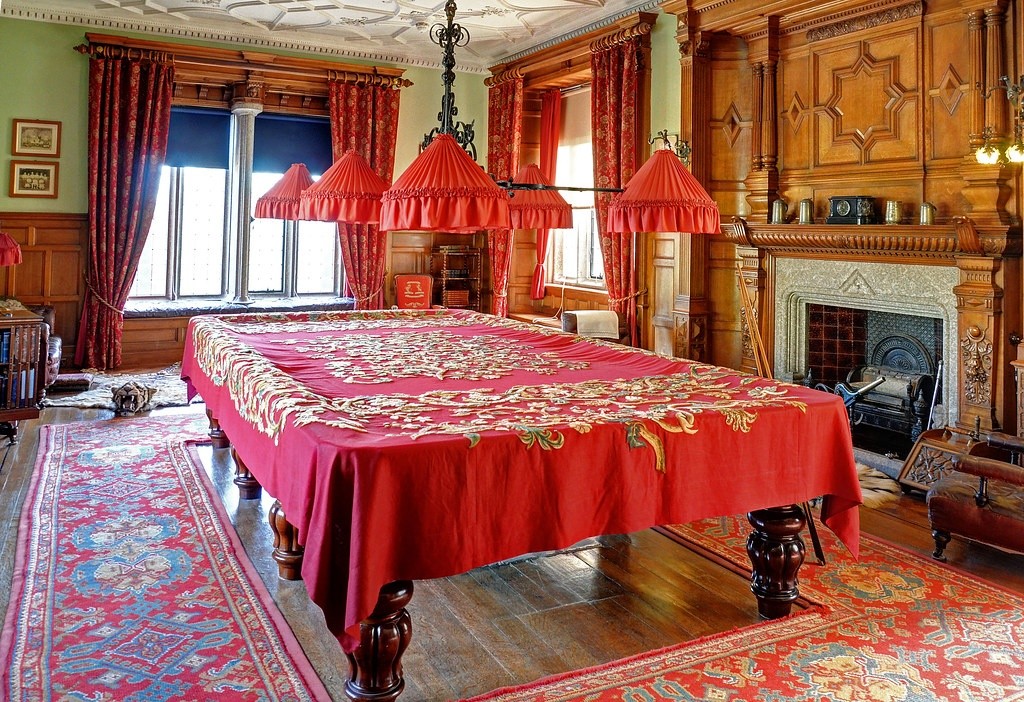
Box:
[389,273,444,309]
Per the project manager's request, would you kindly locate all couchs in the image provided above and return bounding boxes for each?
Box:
[924,431,1024,565]
[26,305,63,387]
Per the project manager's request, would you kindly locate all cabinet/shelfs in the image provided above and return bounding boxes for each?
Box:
[430,246,482,311]
[0,308,47,446]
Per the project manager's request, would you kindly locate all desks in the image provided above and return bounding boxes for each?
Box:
[180,306,863,702]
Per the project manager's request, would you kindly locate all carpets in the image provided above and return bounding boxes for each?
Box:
[39,360,205,417]
[0,413,1023,702]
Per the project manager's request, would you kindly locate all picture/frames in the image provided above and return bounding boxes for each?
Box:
[9,160,59,199]
[12,119,61,158]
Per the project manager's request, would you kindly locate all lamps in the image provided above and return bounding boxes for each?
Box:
[972,74,1023,166]
[254,0,725,238]
[0,233,23,267]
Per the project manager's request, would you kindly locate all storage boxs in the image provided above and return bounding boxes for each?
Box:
[443,288,469,309]
